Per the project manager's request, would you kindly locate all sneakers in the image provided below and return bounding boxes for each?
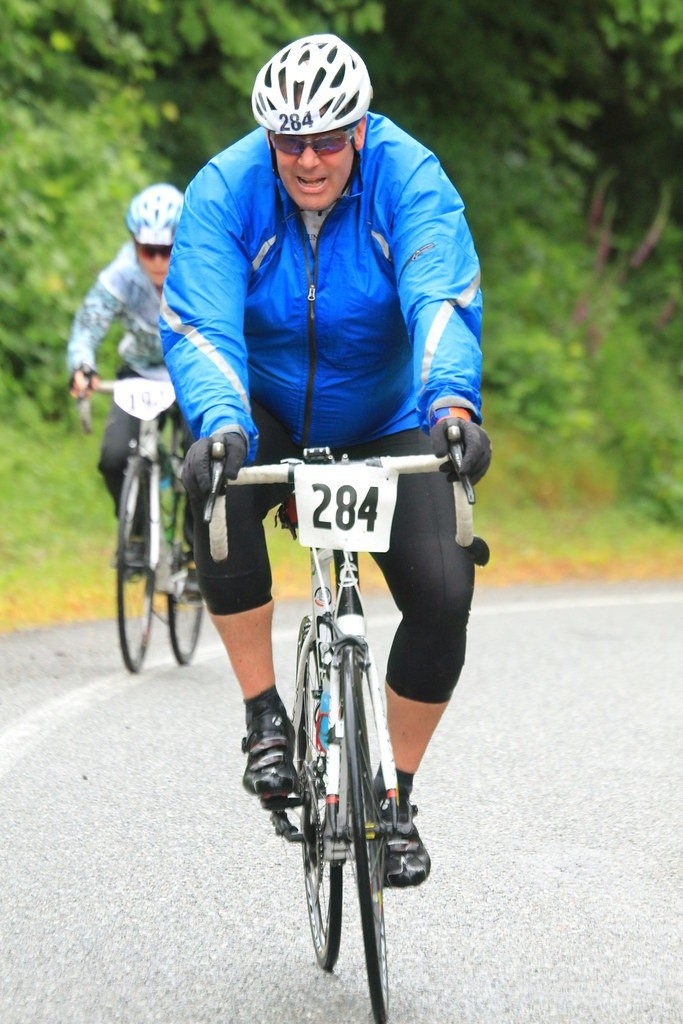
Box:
[241,710,297,797]
[372,786,431,887]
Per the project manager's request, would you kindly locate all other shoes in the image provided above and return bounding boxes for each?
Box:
[113,543,146,571]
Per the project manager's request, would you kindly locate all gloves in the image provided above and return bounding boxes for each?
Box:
[429,414,491,488]
[180,432,246,503]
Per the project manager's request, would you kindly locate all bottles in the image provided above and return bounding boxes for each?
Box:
[318,676,330,750]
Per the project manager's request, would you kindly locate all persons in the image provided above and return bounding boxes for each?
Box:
[66,183,197,602]
[156,34,492,887]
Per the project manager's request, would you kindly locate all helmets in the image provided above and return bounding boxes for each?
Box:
[250,34,373,137]
[125,183,185,248]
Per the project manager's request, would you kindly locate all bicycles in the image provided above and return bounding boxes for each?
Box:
[74,363,204,678]
[184,420,481,1023]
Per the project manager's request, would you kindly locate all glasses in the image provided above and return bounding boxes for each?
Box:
[136,246,175,260]
[268,128,359,155]
